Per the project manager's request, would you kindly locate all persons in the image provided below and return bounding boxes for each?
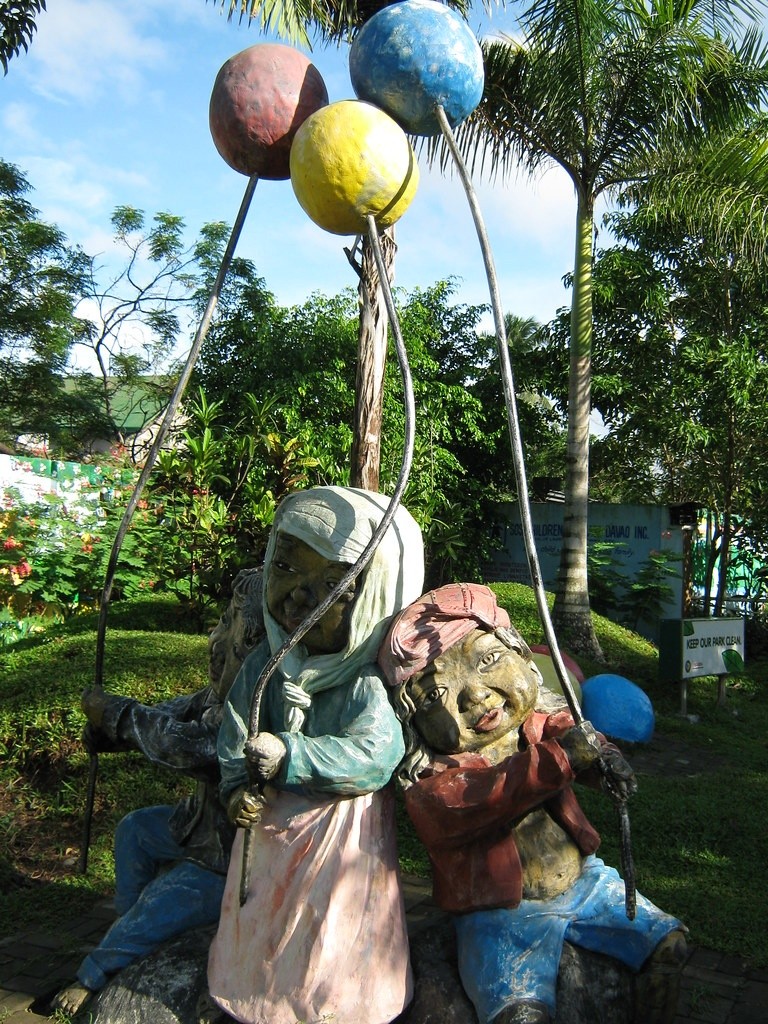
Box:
[380,580,698,1024]
[48,488,427,1018]
[48,570,268,780]
